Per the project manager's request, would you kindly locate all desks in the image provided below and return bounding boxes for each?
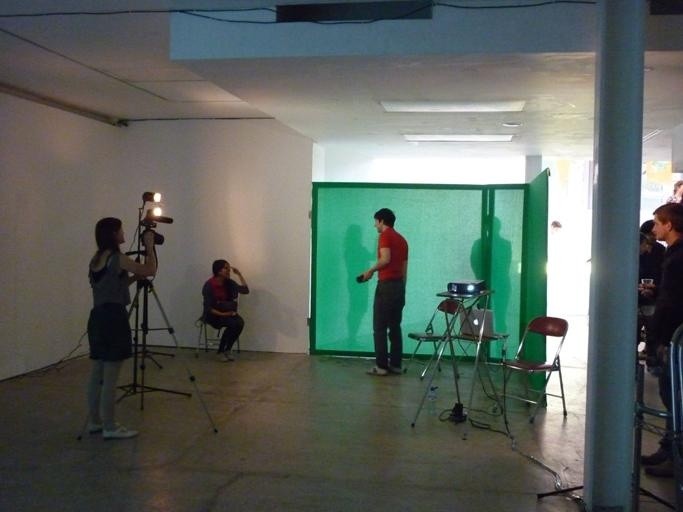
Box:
[411,289,496,428]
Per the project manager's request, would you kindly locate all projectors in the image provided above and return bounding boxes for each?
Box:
[449,278,484,295]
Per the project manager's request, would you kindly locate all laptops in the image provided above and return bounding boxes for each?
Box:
[461,308,509,340]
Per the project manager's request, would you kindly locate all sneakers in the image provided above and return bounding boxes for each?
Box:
[216,350,233,362]
[366,363,401,376]
[641,448,675,475]
[90,425,137,439]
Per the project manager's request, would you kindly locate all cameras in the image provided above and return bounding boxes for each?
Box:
[140,228,165,245]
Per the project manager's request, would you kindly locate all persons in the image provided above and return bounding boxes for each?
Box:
[638,233,653,349]
[88,217,156,438]
[664,179,683,204]
[641,220,664,378]
[362,209,408,376]
[642,204,682,477]
[203,261,249,363]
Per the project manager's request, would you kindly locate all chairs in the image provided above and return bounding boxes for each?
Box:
[493,316,568,425]
[195,291,241,358]
[403,299,464,379]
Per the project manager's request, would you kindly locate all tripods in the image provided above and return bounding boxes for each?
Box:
[76,281,218,440]
[124,283,175,369]
[113,284,192,407]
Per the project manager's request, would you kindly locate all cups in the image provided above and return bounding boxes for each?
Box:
[641,279,653,290]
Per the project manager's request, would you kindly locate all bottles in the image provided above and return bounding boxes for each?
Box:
[428,387,437,416]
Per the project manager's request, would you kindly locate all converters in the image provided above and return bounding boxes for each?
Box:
[454,402,463,417]
